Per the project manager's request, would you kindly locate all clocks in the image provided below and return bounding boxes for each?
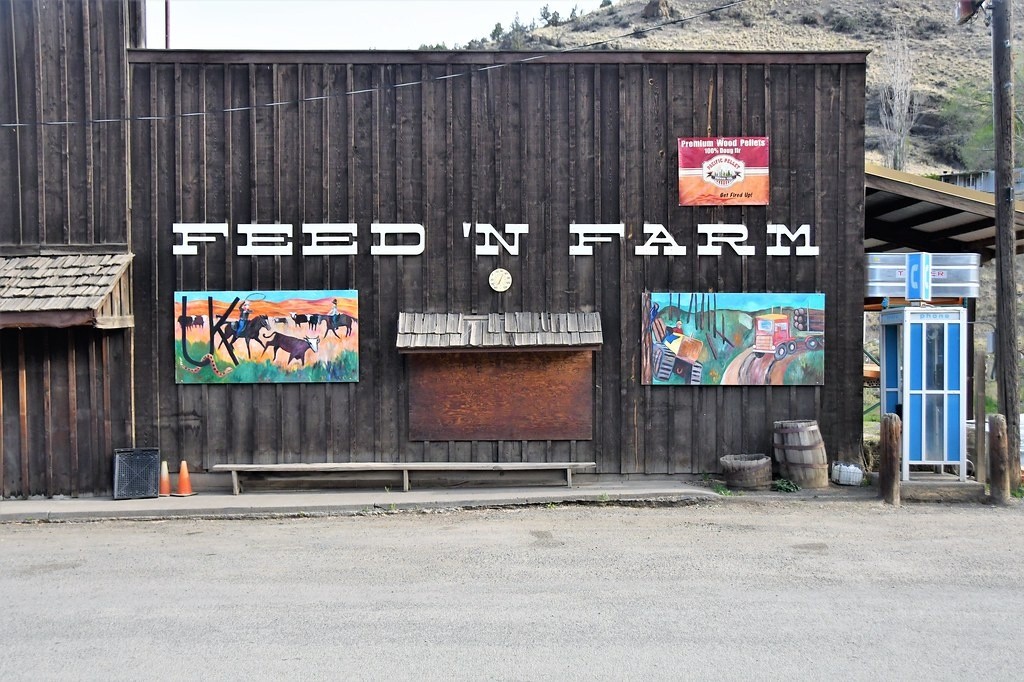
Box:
[489,268,512,292]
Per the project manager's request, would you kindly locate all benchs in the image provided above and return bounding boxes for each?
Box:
[213,462,596,494]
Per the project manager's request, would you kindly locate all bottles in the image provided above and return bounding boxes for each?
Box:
[830,463,863,485]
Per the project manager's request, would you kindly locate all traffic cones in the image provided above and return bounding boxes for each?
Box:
[171,461,198,497]
[159,461,172,496]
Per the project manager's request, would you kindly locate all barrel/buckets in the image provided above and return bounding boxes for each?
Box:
[772,420,828,487]
